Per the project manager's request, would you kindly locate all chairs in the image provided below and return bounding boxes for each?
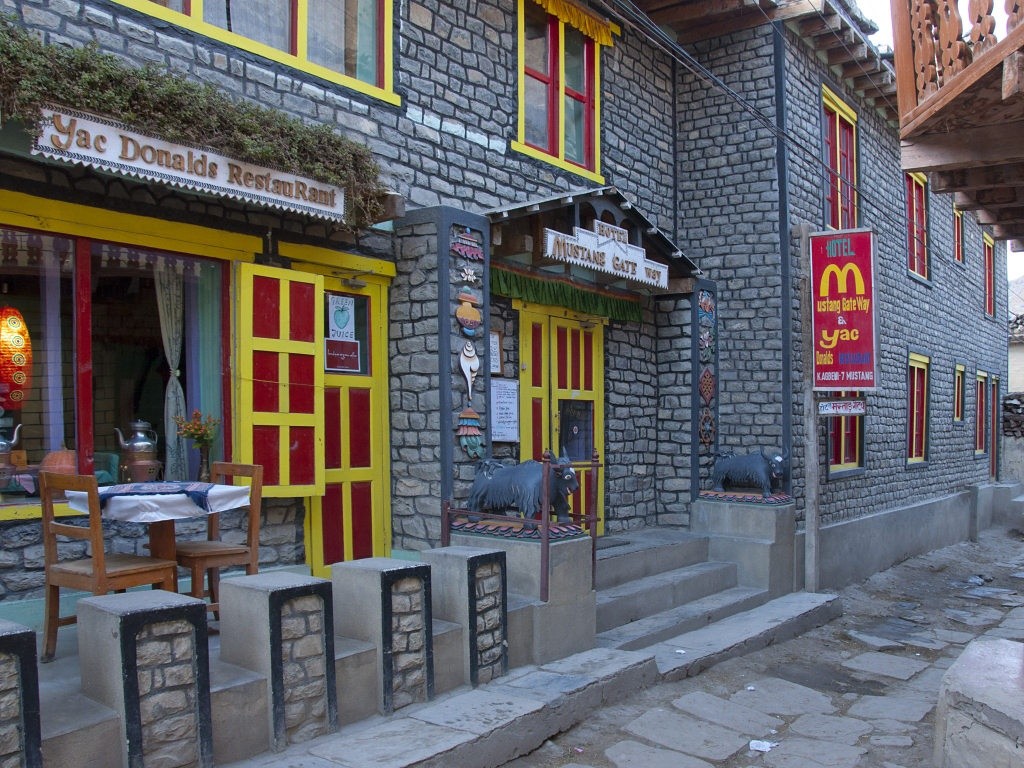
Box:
[142,461,263,612]
[41,472,178,663]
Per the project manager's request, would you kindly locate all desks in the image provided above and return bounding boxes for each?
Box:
[63,481,251,595]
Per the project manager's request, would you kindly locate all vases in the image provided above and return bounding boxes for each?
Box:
[197,443,211,482]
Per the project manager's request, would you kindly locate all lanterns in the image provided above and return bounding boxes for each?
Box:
[0,305,33,412]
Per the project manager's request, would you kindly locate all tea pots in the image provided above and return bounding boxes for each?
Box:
[0,422,24,453]
[113,419,159,459]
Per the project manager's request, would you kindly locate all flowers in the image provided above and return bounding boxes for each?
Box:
[172,409,222,448]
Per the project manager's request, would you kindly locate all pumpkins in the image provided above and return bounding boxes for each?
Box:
[39,442,76,475]
